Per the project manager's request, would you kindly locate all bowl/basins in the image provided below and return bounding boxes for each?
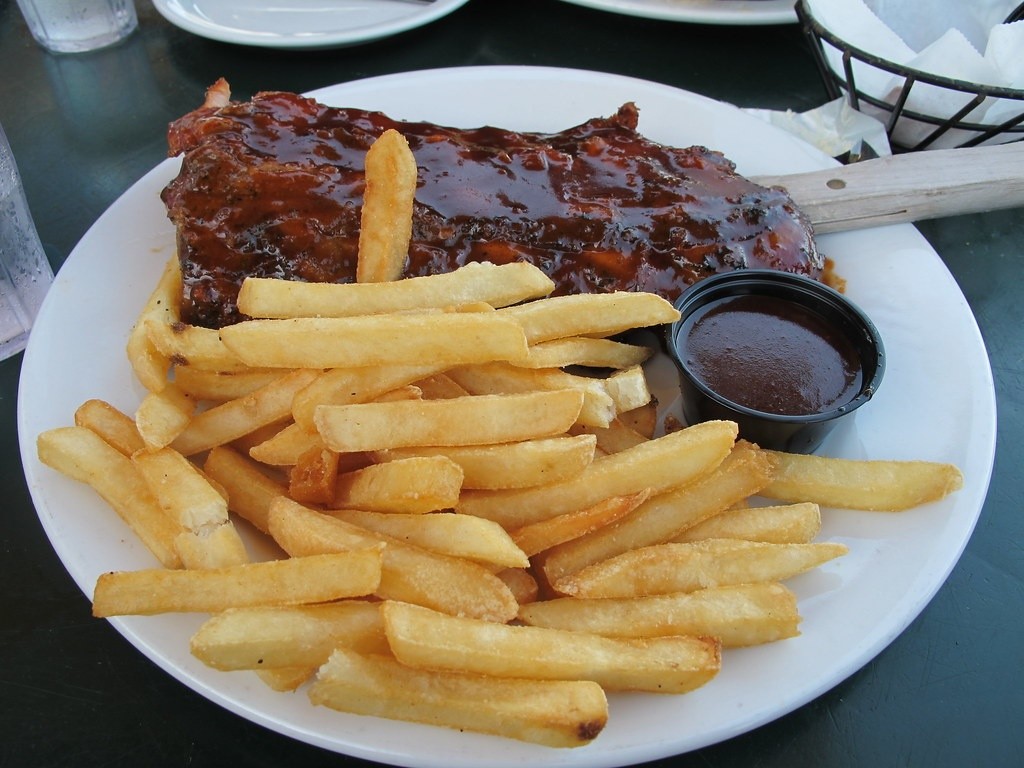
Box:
[664,268,888,455]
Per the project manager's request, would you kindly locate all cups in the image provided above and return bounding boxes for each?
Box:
[0,126,56,363]
[16,0,139,54]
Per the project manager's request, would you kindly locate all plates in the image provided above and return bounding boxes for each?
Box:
[152,0,809,49]
[17,64,996,768]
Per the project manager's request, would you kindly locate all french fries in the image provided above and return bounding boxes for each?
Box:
[36,130,965,748]
